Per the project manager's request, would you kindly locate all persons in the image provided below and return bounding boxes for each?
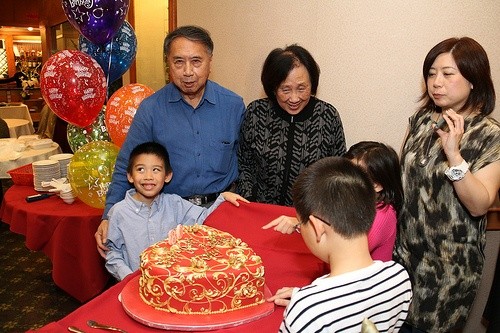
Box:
[267,156,412,333]
[237,43,347,207]
[104,142,251,283]
[262,141,404,262]
[94,24,246,260]
[391,37,499,333]
[8,63,42,89]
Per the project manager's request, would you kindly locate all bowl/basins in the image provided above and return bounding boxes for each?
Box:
[52,178,76,204]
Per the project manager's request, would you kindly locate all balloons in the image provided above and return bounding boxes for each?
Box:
[79,20,138,83]
[105,83,155,147]
[39,50,107,133]
[61,0,130,48]
[67,105,114,153]
[67,141,121,208]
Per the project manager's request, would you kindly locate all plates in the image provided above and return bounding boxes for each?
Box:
[32,160,61,191]
[49,153,74,179]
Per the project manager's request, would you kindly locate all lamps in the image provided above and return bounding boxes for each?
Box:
[0,36,8,77]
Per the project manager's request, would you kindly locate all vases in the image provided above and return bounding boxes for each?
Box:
[23,83,35,101]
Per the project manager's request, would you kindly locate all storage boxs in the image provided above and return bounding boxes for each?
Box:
[7,162,34,187]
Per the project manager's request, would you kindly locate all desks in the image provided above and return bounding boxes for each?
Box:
[0,138,62,179]
[0,184,116,303]
[2,119,33,138]
[0,103,36,135]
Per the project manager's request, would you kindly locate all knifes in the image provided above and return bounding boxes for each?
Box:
[26,192,60,201]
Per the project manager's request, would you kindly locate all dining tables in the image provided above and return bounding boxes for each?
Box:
[26,200,327,333]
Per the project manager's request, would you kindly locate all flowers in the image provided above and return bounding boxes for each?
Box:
[19,55,42,98]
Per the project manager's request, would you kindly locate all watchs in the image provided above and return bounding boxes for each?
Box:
[445,159,469,181]
